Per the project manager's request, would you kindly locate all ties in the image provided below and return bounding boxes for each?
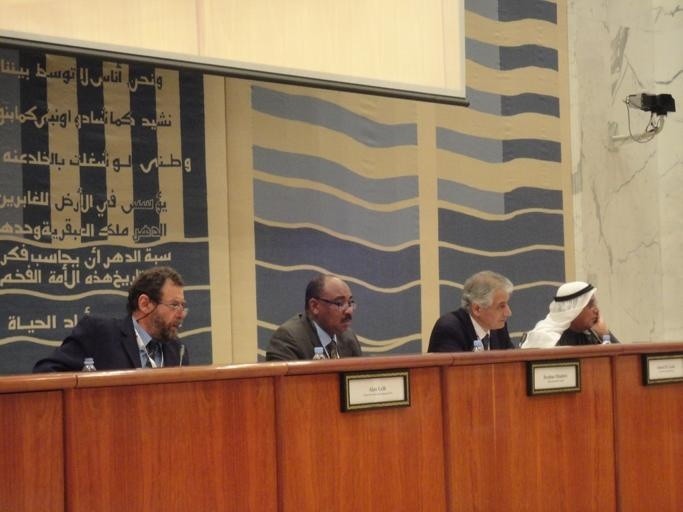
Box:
[152,344,161,367]
[482,334,489,349]
[328,341,338,358]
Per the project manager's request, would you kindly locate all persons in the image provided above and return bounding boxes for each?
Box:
[31,266,190,373]
[427,270,515,354]
[520,281,621,350]
[266,274,363,362]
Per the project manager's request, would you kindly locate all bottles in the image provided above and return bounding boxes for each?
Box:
[601,335,612,344]
[313,345,325,360]
[81,356,96,371]
[471,340,485,351]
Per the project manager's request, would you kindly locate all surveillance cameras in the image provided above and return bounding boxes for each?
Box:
[626,93,677,115]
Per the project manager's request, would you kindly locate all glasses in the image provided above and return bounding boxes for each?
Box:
[314,296,357,311]
